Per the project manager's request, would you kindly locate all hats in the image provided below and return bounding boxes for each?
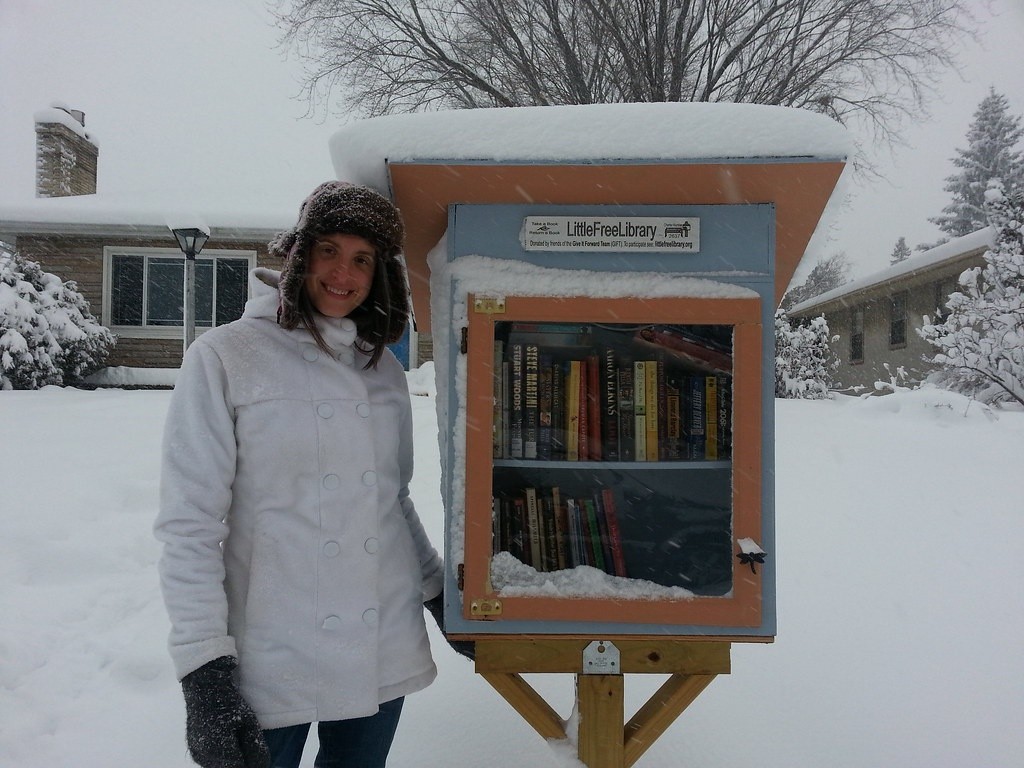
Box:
[269,180,408,348]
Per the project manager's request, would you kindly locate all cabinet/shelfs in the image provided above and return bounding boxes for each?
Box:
[457,292,766,625]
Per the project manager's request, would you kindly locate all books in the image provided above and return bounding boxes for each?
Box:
[493,322,732,462]
[494,488,626,577]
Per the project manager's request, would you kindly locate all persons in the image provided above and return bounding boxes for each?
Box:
[155,181,475,768]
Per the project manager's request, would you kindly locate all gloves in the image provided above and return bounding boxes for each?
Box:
[173,654,272,768]
[427,590,478,664]
